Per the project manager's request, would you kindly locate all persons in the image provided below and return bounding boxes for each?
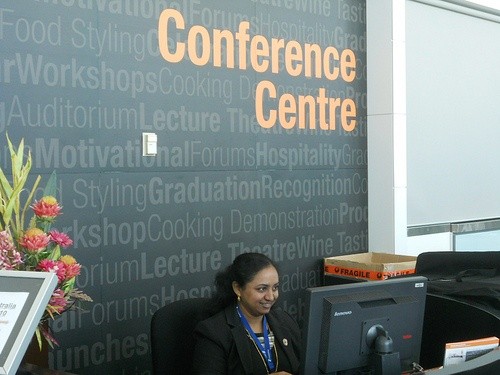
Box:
[193,253,304,375]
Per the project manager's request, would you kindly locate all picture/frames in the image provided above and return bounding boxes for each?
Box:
[0,270,57,375]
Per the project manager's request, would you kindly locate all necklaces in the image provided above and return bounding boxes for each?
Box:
[243,324,278,375]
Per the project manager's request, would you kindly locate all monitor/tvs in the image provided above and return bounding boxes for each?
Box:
[299,276,428,373]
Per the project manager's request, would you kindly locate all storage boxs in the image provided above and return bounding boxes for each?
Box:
[323,250,418,282]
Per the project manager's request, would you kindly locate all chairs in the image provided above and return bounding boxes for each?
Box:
[150,297,230,375]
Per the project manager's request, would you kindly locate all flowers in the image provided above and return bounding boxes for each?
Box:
[0,127,95,352]
